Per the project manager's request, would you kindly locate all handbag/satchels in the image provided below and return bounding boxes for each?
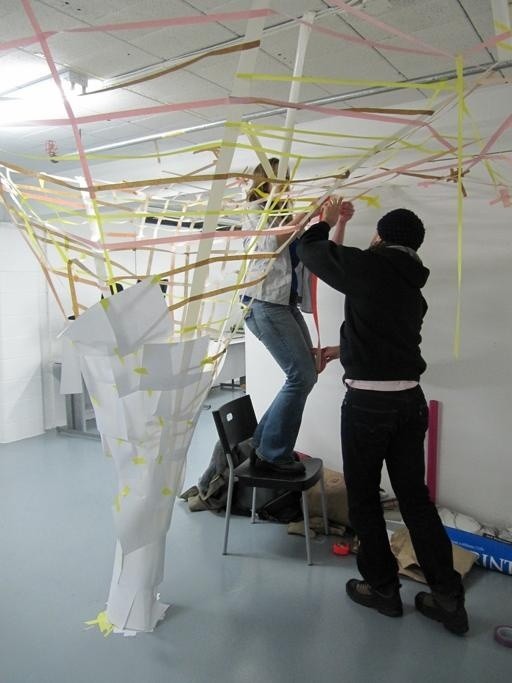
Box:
[198,439,305,523]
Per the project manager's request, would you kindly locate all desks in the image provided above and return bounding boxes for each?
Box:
[40,356,107,439]
[201,329,246,410]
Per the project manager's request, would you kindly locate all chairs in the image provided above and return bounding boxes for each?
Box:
[212,394,328,564]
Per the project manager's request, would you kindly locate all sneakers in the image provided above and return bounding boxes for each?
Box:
[415,591,469,633]
[253,453,304,474]
[346,579,403,617]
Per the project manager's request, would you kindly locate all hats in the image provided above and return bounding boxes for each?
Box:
[377,208,425,251]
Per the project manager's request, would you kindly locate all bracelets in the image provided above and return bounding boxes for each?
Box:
[303,210,310,216]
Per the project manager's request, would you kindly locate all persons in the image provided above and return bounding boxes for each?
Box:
[294,195,474,638]
[238,158,356,475]
